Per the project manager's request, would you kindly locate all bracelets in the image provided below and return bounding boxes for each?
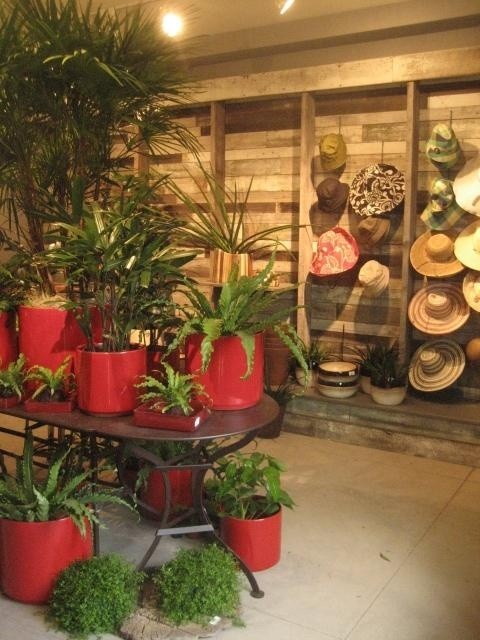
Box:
[315,360,359,398]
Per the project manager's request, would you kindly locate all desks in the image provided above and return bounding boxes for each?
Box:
[1,395,279,595]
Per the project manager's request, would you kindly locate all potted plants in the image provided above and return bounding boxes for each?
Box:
[0,1,412,640]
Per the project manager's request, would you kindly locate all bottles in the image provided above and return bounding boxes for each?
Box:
[309,226,360,278]
[452,219,480,313]
[420,122,479,229]
[351,162,406,216]
[316,177,349,213]
[356,259,391,300]
[409,230,463,279]
[358,217,393,247]
[408,339,465,394]
[408,283,470,335]
[319,132,348,172]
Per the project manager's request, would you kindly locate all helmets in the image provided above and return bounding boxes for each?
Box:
[349,162,406,216]
